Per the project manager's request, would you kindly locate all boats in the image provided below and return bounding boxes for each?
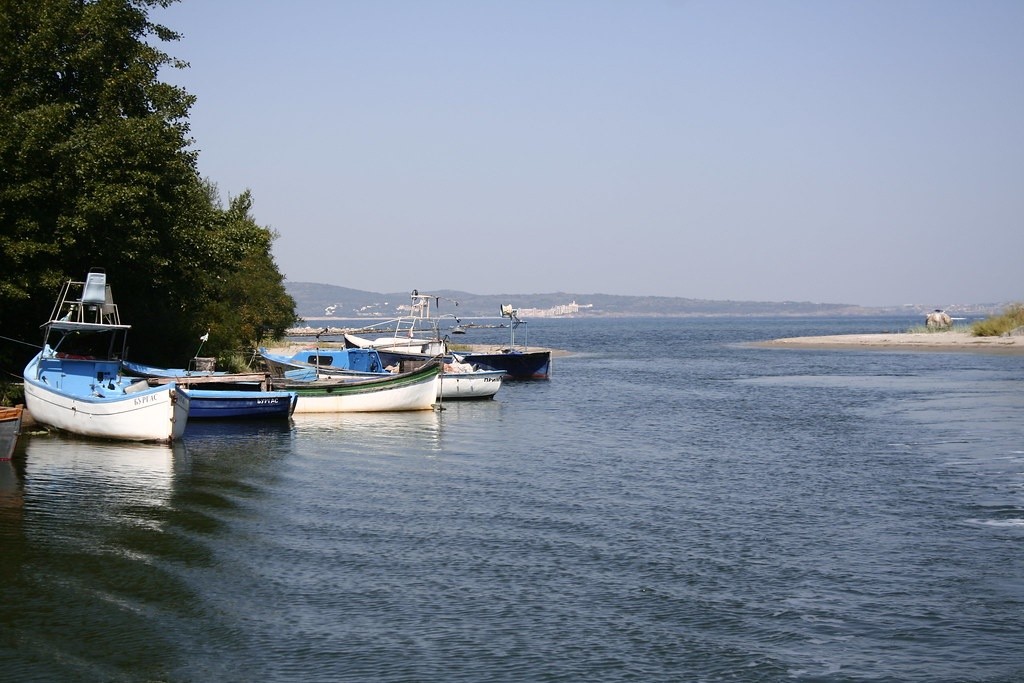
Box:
[23,267,192,444]
[0,403,24,461]
[259,343,510,401]
[111,352,445,417]
[343,289,553,382]
